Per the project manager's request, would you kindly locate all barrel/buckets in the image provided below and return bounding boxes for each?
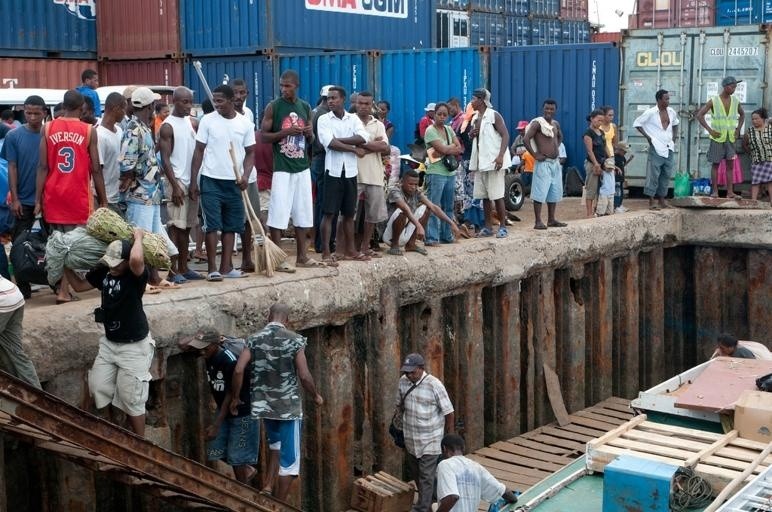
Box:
[693,178,711,194]
[672,171,691,197]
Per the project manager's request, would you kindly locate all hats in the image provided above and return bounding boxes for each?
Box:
[514,120,528,130]
[98,239,131,268]
[617,141,632,153]
[399,353,425,372]
[604,158,615,170]
[473,87,494,109]
[722,76,743,87]
[320,85,336,98]
[424,102,437,113]
[124,86,162,108]
[188,325,220,350]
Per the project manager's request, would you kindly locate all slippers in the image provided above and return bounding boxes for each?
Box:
[246,469,272,495]
[277,248,383,273]
[547,219,568,227]
[649,202,674,210]
[145,262,257,295]
[711,193,742,200]
[386,226,508,256]
[55,293,81,307]
[533,222,548,230]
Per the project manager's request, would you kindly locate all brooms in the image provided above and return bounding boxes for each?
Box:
[230,142,265,273]
[229,150,287,278]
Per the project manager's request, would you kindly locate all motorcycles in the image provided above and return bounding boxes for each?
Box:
[396,147,525,210]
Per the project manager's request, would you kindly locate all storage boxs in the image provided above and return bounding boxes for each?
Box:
[346,471,416,511]
[717,389,772,446]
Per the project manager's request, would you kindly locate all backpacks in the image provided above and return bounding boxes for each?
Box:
[563,165,585,196]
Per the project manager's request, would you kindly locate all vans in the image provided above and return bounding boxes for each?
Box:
[1,83,190,128]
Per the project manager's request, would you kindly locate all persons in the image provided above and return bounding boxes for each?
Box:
[395,351,454,512]
[49,225,157,437]
[633,88,681,211]
[0,274,46,391]
[742,107,772,202]
[710,332,757,359]
[593,157,616,216]
[697,76,745,199]
[614,140,635,212]
[179,327,259,488]
[435,430,518,512]
[597,105,617,203]
[231,301,324,501]
[582,108,611,218]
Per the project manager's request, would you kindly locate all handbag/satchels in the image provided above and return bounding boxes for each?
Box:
[691,178,711,195]
[9,228,52,286]
[674,169,691,196]
[388,407,405,450]
[445,154,459,172]
